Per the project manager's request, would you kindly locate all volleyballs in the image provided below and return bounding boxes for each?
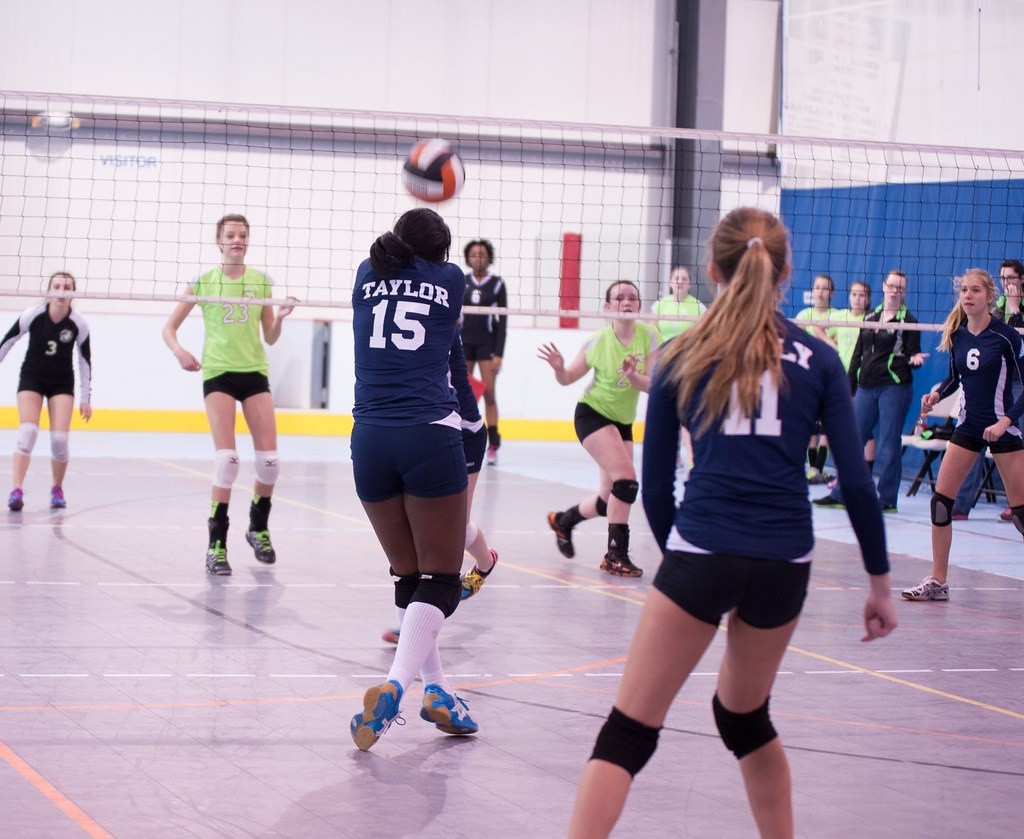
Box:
[402,136,468,203]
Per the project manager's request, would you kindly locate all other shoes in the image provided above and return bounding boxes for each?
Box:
[951,505,968,520]
[997,508,1014,524]
[806,468,835,483]
[487,445,496,464]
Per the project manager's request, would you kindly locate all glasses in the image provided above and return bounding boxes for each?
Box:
[884,281,905,293]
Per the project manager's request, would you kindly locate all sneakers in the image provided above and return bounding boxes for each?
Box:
[419,684,478,734]
[600,553,643,578]
[350,679,407,751]
[245,528,275,563]
[459,549,498,600]
[50,487,66,506]
[206,541,232,574]
[9,490,23,510]
[382,628,399,643]
[878,501,897,514]
[812,496,847,511]
[901,578,950,601]
[548,509,574,559]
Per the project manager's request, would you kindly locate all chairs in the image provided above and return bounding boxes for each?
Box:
[901,382,1006,509]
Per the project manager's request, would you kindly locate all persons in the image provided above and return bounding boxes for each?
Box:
[901,268,1024,601]
[650,265,707,342]
[164,214,294,576]
[537,280,664,578]
[812,270,930,513]
[0,273,92,511]
[796,274,874,386]
[351,208,479,751]
[990,260,1024,341]
[571,207,897,839]
[462,239,508,465]
[382,322,499,643]
